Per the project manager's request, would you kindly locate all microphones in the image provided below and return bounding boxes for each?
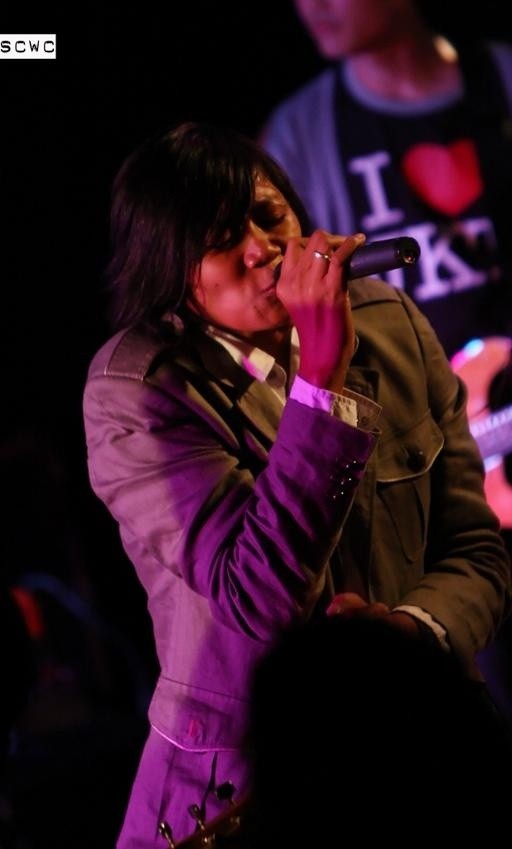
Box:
[272,235,422,298]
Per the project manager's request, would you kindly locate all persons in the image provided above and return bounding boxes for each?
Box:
[256,0,511,718]
[79,119,512,847]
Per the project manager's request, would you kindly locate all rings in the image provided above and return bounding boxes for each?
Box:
[311,250,331,262]
